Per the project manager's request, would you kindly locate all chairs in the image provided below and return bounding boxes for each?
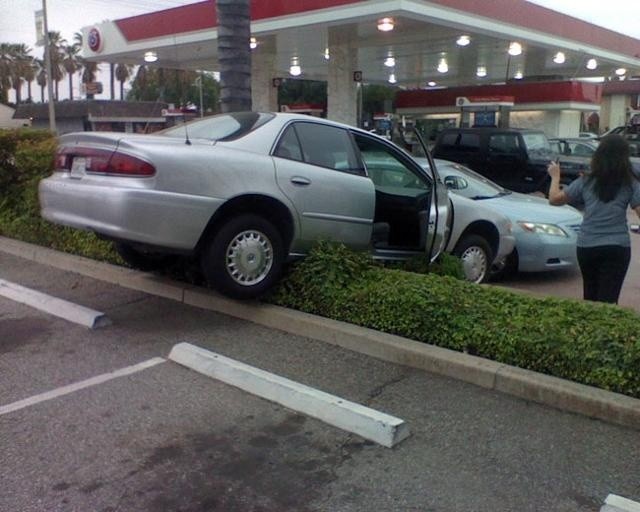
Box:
[309,144,335,169]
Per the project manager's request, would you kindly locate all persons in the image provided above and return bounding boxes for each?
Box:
[421,134,439,158]
[545,133,640,304]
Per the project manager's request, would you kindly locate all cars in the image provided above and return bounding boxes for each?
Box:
[35,111,516,302]
[334,156,585,282]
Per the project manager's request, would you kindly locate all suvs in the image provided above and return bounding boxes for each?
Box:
[541,126,640,183]
[431,127,589,200]
